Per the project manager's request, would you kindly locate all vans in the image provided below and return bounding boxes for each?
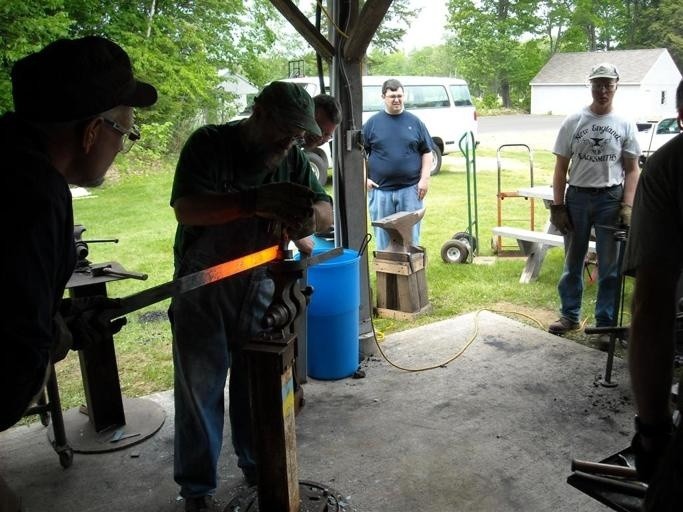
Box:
[228,75,478,187]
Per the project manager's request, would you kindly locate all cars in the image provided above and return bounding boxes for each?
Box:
[636,114,680,169]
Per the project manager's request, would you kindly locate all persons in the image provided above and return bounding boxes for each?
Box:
[550,63,642,346]
[304,94,343,149]
[363,79,433,251]
[0,36,158,432]
[621,79,683,512]
[168,82,333,512]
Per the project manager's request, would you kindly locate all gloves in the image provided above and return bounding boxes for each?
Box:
[550,203,573,235]
[241,182,318,231]
[58,295,126,351]
[617,203,632,227]
[44,317,73,364]
[631,426,678,487]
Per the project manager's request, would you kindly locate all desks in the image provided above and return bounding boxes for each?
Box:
[518,183,570,284]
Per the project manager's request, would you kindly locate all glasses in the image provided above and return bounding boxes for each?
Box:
[591,83,616,90]
[271,125,304,146]
[323,134,333,143]
[100,115,141,154]
[385,94,402,98]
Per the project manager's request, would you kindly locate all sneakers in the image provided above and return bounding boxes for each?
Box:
[185,494,215,512]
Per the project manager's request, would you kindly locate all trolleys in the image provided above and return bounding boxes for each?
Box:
[490,143,534,257]
[440,130,480,264]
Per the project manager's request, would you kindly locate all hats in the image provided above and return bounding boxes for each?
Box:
[12,37,157,124]
[255,81,322,137]
[589,64,620,82]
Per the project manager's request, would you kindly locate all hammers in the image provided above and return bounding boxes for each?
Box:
[90,264,149,280]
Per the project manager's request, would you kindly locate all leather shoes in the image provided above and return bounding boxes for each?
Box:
[598,334,613,351]
[548,317,580,336]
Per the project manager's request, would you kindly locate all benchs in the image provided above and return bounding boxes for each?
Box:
[494,226,597,254]
